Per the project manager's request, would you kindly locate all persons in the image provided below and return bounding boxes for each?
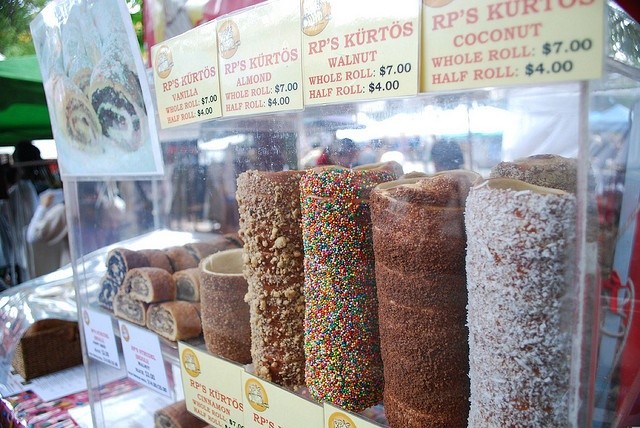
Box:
[27,180,137,268]
[4,140,47,196]
[317,138,360,167]
[426,139,464,175]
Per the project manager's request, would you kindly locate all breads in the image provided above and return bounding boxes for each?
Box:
[488,153,575,191]
[98,233,251,363]
[45,66,150,150]
[300,162,404,415]
[155,400,213,426]
[462,179,579,428]
[237,170,306,386]
[369,169,481,428]
